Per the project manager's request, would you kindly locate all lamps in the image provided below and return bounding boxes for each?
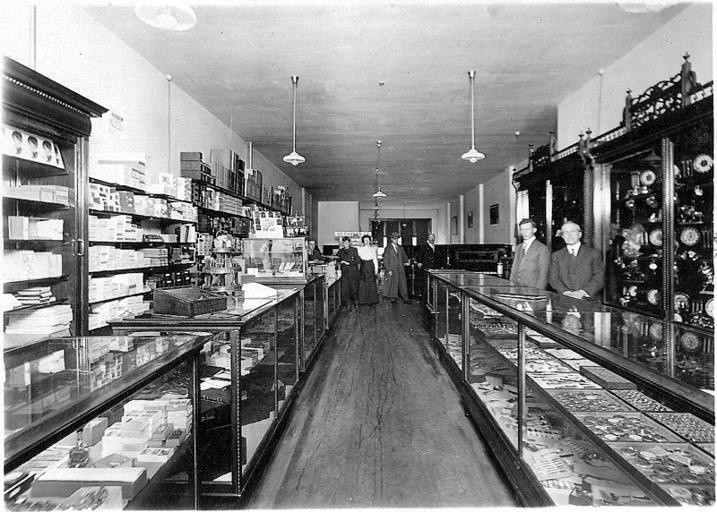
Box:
[280,70,308,175]
[458,66,488,169]
[367,137,390,232]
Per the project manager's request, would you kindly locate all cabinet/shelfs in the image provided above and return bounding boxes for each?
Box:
[507,138,585,250]
[1,56,110,358]
[423,270,531,389]
[86,173,199,325]
[109,285,305,506]
[185,170,295,275]
[423,268,715,504]
[666,79,713,386]
[4,332,213,511]
[461,285,714,507]
[241,270,346,382]
[589,109,673,381]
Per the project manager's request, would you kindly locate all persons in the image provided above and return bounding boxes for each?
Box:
[334,236,362,315]
[547,221,606,304]
[355,233,379,307]
[507,217,551,290]
[550,293,602,338]
[413,233,444,304]
[381,231,413,305]
[507,280,547,319]
[303,237,324,261]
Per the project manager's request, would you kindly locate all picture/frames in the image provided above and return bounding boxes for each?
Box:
[450,216,459,236]
